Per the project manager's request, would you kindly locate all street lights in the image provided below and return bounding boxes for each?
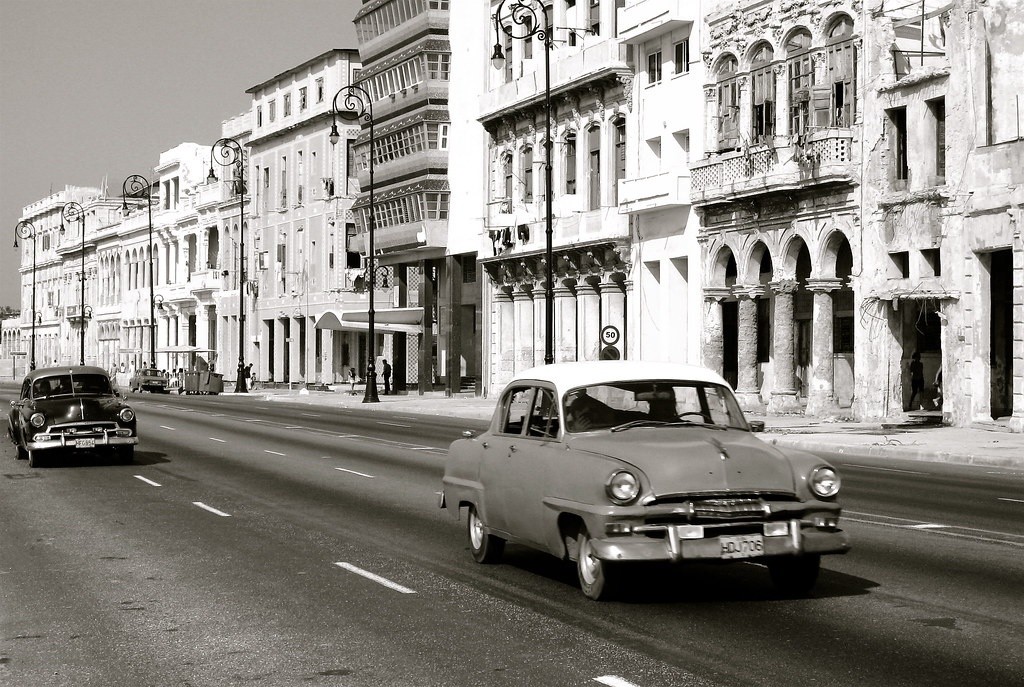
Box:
[491,0,557,417]
[122,175,164,376]
[329,86,389,403]
[59,202,93,386]
[13,221,42,372]
[206,138,249,393]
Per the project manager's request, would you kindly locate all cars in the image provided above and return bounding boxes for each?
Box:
[128,368,167,393]
[8,366,140,468]
[438,360,851,601]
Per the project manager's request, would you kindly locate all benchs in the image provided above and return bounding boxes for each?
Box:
[333,381,418,390]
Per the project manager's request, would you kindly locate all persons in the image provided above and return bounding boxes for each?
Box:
[121,363,126,373]
[569,398,613,432]
[251,373,256,387]
[348,368,357,390]
[244,363,253,393]
[143,362,147,368]
[111,363,117,385]
[933,363,942,407]
[908,352,924,409]
[129,361,135,372]
[381,360,391,395]
[650,386,692,423]
[51,360,59,367]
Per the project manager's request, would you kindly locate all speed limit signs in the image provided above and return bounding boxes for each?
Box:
[600,326,620,345]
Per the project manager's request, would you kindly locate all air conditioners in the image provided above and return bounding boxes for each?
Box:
[66,304,79,316]
